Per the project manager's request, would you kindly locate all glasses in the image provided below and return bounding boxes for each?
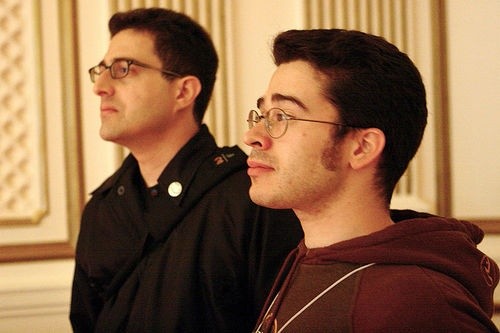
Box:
[89,57,184,84]
[246,107,345,139]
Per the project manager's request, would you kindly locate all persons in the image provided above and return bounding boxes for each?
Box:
[243,28,500,333]
[68,7,305,333]
